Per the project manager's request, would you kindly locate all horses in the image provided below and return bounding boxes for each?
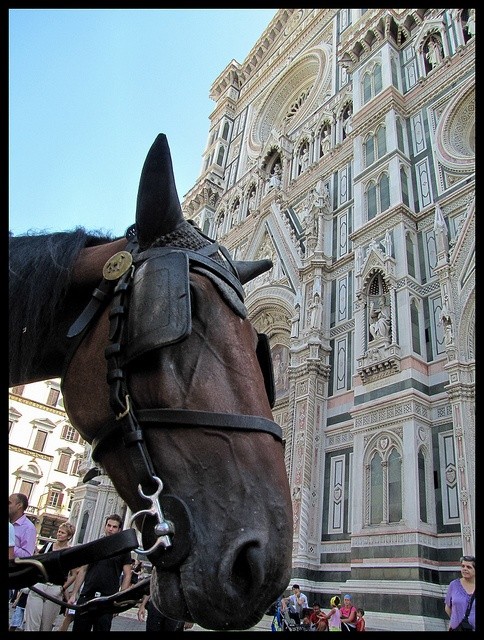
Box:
[9,133,294,631]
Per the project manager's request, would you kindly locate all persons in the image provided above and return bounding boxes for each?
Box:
[444,557,476,630]
[25,521,74,632]
[355,608,368,632]
[368,294,391,338]
[138,590,194,636]
[9,587,28,631]
[309,603,326,631]
[7,493,37,631]
[130,558,135,568]
[130,560,142,584]
[9,523,17,570]
[343,592,353,630]
[282,585,311,611]
[327,596,343,631]
[70,515,133,634]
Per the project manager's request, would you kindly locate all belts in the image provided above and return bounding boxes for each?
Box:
[81,590,111,597]
[37,580,63,586]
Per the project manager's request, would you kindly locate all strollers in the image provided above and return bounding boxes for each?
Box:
[273,602,304,631]
